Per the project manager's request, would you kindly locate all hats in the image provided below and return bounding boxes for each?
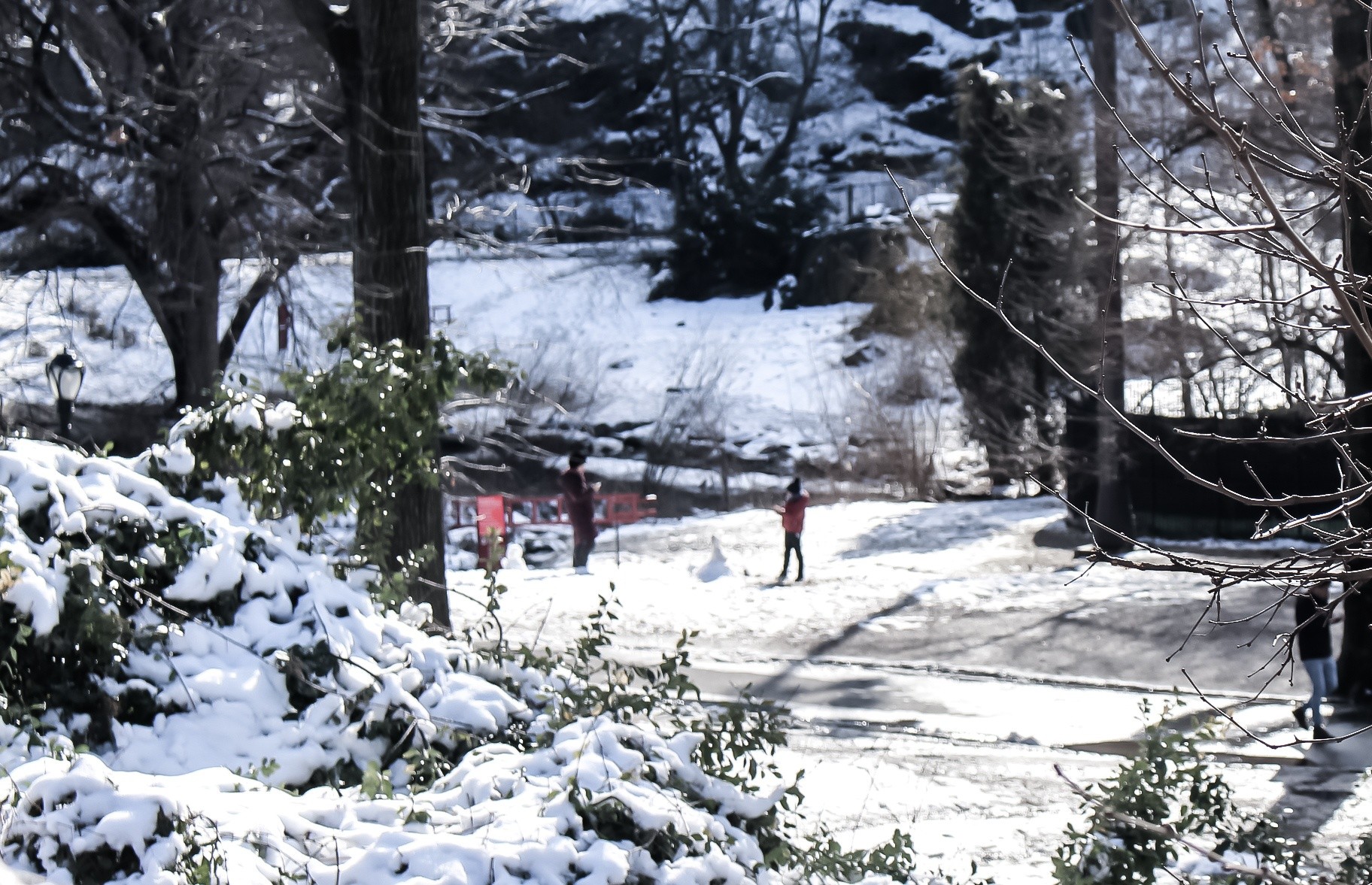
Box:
[787,478,801,495]
[569,454,587,468]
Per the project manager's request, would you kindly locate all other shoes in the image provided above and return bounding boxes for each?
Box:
[1292,707,1309,729]
[573,566,590,575]
[1313,728,1336,739]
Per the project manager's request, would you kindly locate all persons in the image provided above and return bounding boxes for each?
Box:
[1292,581,1344,739]
[773,476,810,581]
[562,450,601,575]
